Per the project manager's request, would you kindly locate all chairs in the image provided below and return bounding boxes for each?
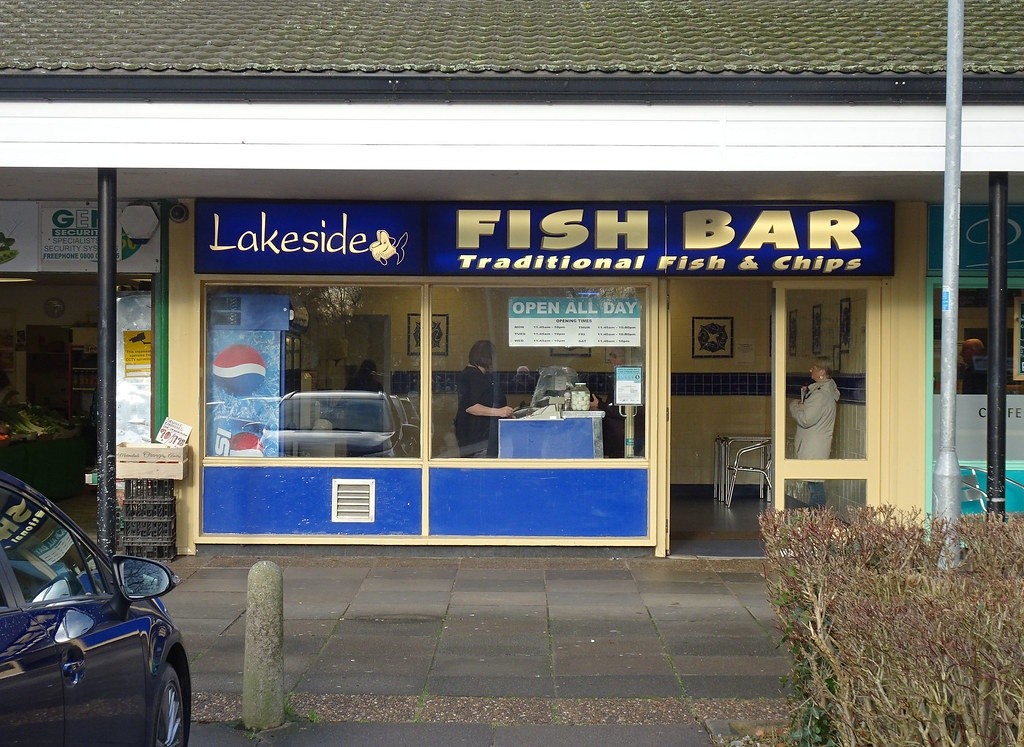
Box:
[725,439,771,508]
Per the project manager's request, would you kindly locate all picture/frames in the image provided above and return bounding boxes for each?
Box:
[788,309,797,356]
[692,316,734,358]
[550,347,591,357]
[812,304,822,354]
[407,313,448,356]
[770,314,772,357]
[839,297,852,354]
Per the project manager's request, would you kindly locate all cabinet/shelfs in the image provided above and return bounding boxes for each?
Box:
[70,327,98,418]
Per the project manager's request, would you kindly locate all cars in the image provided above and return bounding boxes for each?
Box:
[244,389,421,460]
[0,470,192,747]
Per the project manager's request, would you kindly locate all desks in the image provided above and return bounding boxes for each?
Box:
[712,434,771,504]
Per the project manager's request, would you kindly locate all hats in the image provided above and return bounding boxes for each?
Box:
[962,339,984,355]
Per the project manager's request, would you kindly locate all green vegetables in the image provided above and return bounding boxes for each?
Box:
[13,405,59,436]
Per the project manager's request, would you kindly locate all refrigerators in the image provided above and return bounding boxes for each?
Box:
[205,289,310,461]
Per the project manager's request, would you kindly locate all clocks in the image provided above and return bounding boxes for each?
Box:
[44,298,65,319]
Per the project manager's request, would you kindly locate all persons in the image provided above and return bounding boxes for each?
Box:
[959,339,987,395]
[322,360,383,457]
[454,341,513,458]
[589,347,645,459]
[788,356,839,509]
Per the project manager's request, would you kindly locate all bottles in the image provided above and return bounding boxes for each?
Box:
[570,382,590,411]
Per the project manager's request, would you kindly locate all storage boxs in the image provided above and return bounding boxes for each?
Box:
[121,515,176,541]
[116,442,189,480]
[122,496,176,520]
[124,479,174,500]
[123,538,177,563]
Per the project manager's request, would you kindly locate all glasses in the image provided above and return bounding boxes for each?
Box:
[609,352,624,359]
